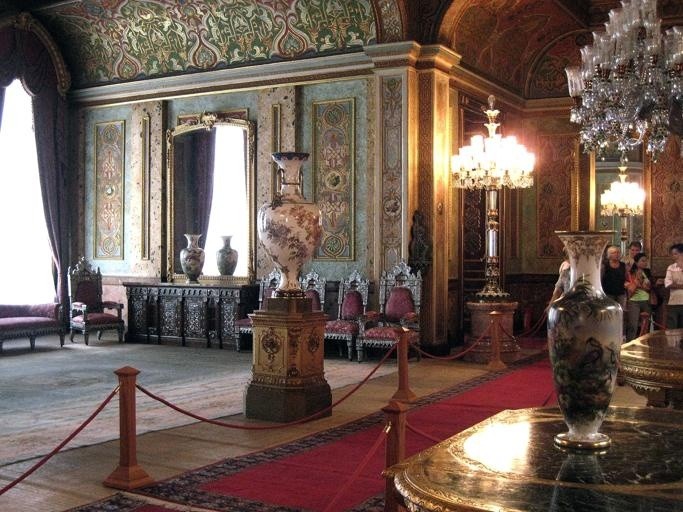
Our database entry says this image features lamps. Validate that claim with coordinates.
[598,166,648,257]
[447,93,538,303]
[562,0,682,167]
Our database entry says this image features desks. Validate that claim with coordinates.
[376,401,681,512]
[120,281,260,354]
[615,326,682,411]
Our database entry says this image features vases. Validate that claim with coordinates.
[180,232,206,285]
[255,151,324,298]
[545,229,626,457]
[216,235,238,275]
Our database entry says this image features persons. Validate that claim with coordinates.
[542,246,571,310]
[599,240,682,342]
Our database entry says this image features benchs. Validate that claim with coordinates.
[0,301,70,357]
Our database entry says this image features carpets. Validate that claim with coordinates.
[0,328,424,467]
[57,337,558,512]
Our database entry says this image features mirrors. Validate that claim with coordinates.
[586,127,653,270]
[163,106,258,285]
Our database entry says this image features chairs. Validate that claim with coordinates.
[65,254,125,346]
[231,256,424,365]
